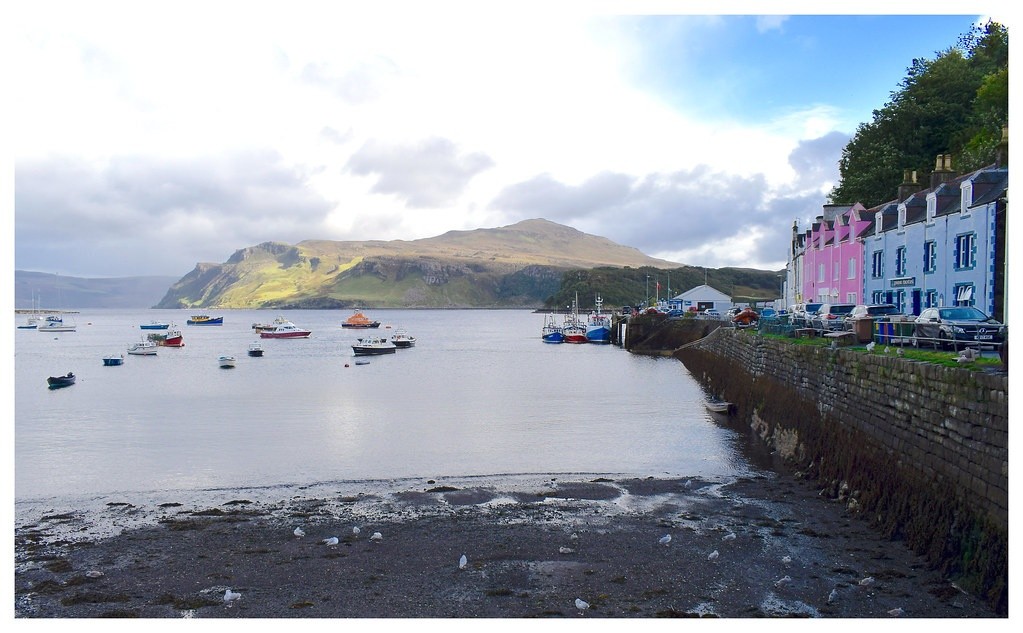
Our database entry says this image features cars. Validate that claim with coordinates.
[727,303,919,346]
[621,305,722,320]
[912,305,1005,351]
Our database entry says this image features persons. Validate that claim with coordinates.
[734,306,741,315]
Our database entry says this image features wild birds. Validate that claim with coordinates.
[86,570,101,577]
[353,526,360,538]
[370,532,383,542]
[828,589,839,602]
[775,555,792,587]
[459,555,467,569]
[224,590,241,608]
[323,537,338,549]
[722,533,737,541]
[888,608,904,616]
[659,534,671,546]
[560,547,571,553]
[575,599,589,615]
[685,480,692,489]
[707,550,718,560]
[858,577,875,586]
[865,341,904,358]
[294,527,305,539]
[571,533,579,544]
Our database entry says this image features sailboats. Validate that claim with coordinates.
[246,340,265,357]
[37,269,79,332]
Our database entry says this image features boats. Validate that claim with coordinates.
[187,314,224,326]
[218,356,236,368]
[350,335,397,355]
[17,286,65,329]
[126,333,158,356]
[340,299,381,329]
[139,320,170,329]
[391,320,417,348]
[102,353,126,365]
[147,320,186,347]
[251,312,312,339]
[540,288,612,345]
[46,371,77,388]
